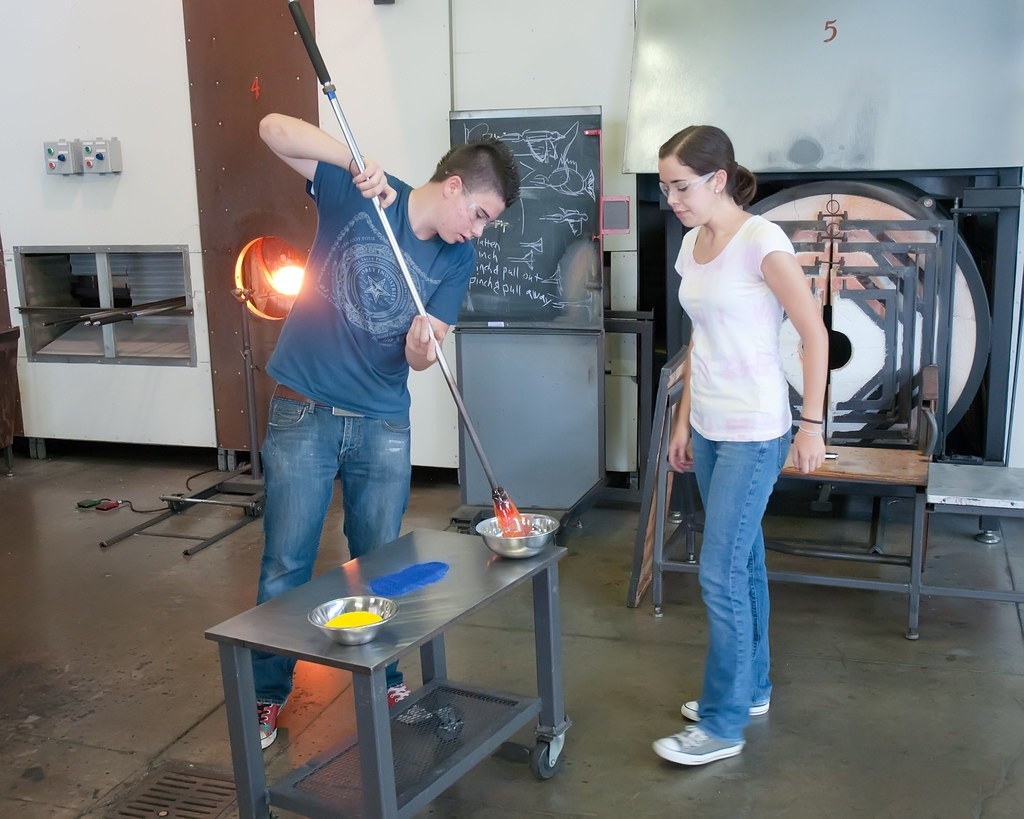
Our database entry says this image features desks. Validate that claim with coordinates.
[202,522,574,817]
[667,434,1024,651]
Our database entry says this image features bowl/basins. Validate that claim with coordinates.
[476,513,561,559]
[308,595,400,645]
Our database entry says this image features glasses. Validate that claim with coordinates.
[658,171,716,199]
[445,170,497,230]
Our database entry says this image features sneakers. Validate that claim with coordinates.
[387,683,432,724]
[653,725,746,766]
[256,702,284,750]
[680,700,770,723]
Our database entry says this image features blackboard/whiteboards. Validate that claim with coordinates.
[446,104,606,338]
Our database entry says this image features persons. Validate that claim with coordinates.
[651,123,831,767]
[251,110,522,751]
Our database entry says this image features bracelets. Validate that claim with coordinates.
[799,426,825,436]
[350,153,365,179]
[800,417,823,424]
